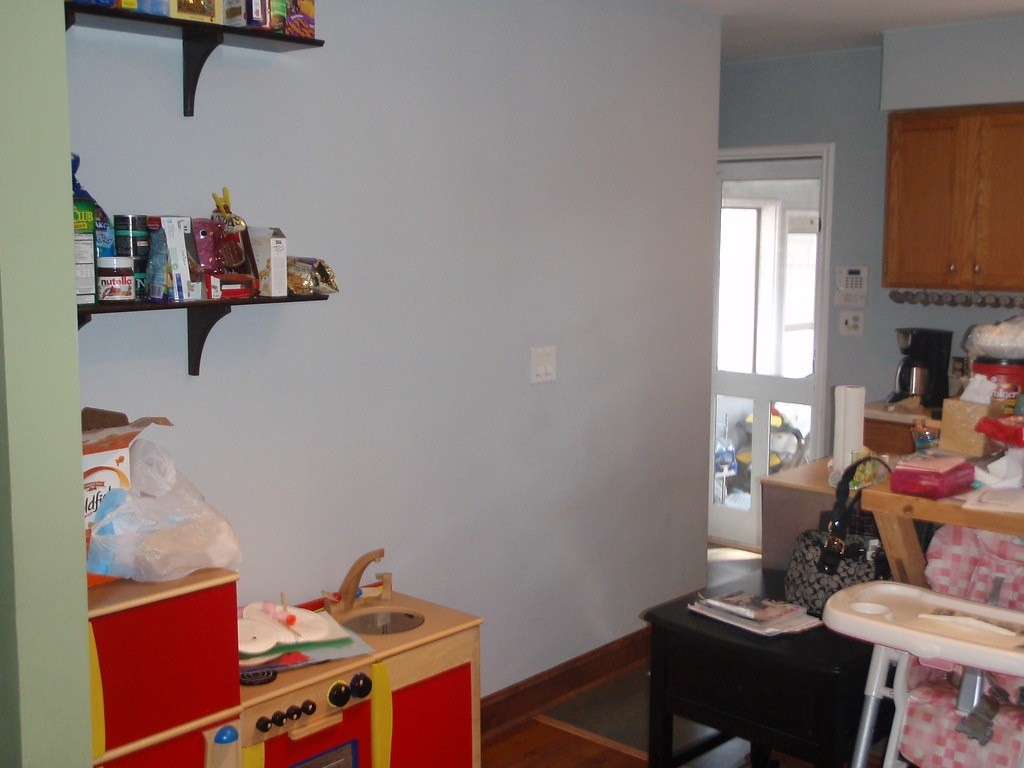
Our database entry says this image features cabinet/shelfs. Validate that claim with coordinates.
[880,102,1024,293]
[64,1,329,376]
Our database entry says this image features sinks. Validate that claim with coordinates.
[335,607,426,635]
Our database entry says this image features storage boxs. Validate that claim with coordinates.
[244,225,287,297]
[171,0,315,38]
[81,407,173,587]
[888,463,976,499]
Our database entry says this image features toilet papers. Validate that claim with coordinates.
[834,384,866,471]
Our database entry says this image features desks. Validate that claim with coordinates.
[639,563,896,768]
[759,457,838,577]
[864,400,938,456]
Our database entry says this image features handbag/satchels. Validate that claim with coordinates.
[784,454,895,617]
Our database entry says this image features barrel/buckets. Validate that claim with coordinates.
[972,356,1024,418]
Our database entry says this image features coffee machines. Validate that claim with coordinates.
[884,326,954,409]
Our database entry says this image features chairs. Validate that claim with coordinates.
[822,487,1023,768]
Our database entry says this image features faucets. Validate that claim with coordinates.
[321,548,386,615]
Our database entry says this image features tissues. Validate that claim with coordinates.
[938,373,1006,457]
